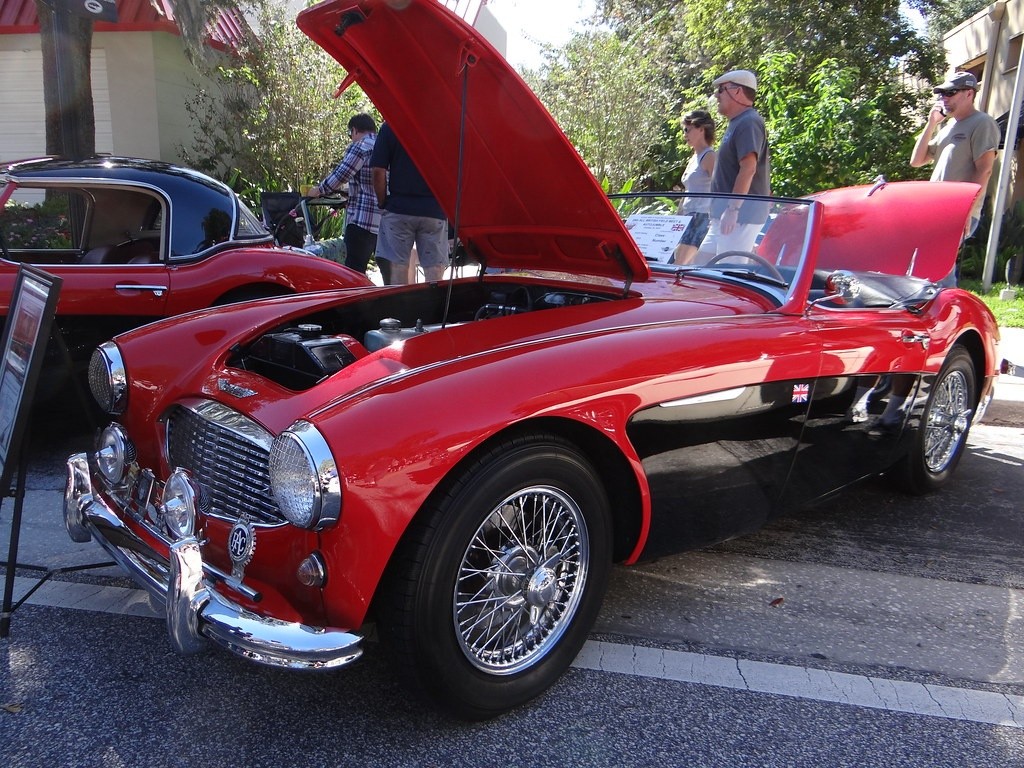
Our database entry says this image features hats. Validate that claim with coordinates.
[713,70,756,90]
[933,72,977,94]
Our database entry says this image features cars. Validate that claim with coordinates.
[63,0,999,720]
[0,153,379,354]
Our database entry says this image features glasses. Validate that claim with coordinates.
[715,86,738,94]
[348,127,355,138]
[683,126,695,134]
[941,89,958,97]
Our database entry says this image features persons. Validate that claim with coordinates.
[692,70,776,272]
[370,118,450,285]
[676,108,717,269]
[908,73,1003,289]
[307,115,392,285]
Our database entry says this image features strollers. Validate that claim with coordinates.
[260,191,348,257]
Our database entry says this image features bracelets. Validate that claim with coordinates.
[727,205,740,211]
[377,201,385,210]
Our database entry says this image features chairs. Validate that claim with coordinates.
[78,231,163,265]
